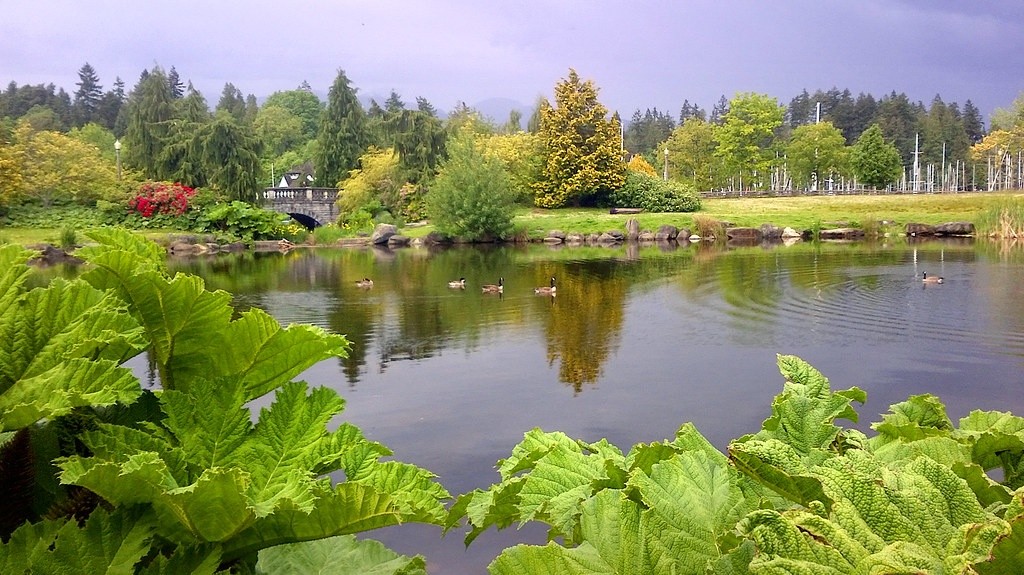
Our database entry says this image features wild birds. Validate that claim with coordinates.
[448,278,466,286]
[354,277,374,287]
[481,276,505,290]
[532,277,557,292]
[922,270,945,282]
